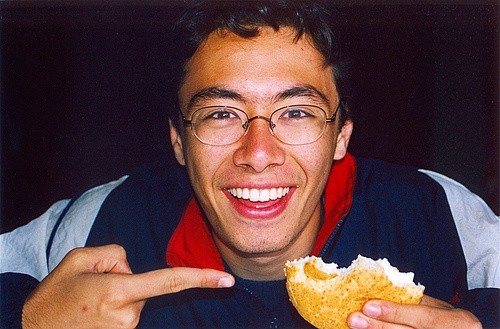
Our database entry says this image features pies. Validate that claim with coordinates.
[283,254,425,329]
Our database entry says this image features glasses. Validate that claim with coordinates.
[174,101,340,146]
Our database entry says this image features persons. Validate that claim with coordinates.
[0,1,500,329]
